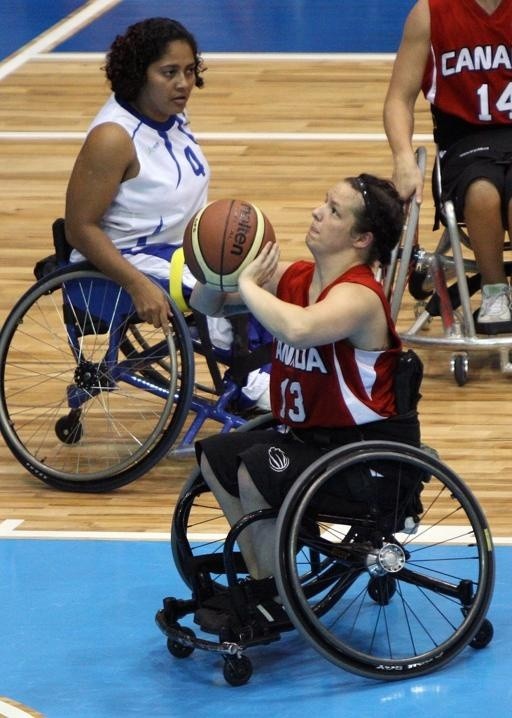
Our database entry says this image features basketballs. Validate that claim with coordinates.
[184,199,276,294]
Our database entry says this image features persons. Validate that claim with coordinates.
[64,17,272,414]
[383,0,512,335]
[189,175,402,634]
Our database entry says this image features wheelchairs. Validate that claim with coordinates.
[375,140,512,389]
[0,213,284,496]
[152,346,497,689]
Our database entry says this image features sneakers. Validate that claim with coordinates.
[476,283,512,335]
[194,574,284,633]
